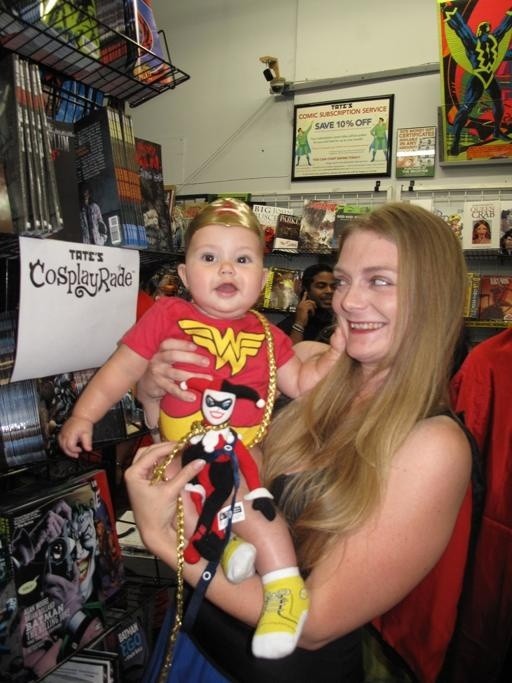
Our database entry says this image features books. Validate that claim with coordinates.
[1,0,176,257]
[419,198,511,323]
[0,295,151,682]
[176,193,380,314]
[142,255,194,304]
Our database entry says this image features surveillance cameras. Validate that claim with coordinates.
[263,68,277,82]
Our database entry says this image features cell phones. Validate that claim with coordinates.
[299,287,310,301]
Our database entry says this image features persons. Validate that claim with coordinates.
[55,197,345,663]
[275,263,337,344]
[120,200,478,681]
[448,321,512,683]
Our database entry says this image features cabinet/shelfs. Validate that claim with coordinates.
[171,185,512,361]
[0,0,193,683]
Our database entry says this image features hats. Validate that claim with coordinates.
[183,196,266,258]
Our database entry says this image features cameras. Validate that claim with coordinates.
[44,535,76,589]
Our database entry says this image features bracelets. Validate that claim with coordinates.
[140,408,160,437]
[290,321,304,336]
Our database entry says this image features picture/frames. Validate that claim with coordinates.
[291,93,395,181]
